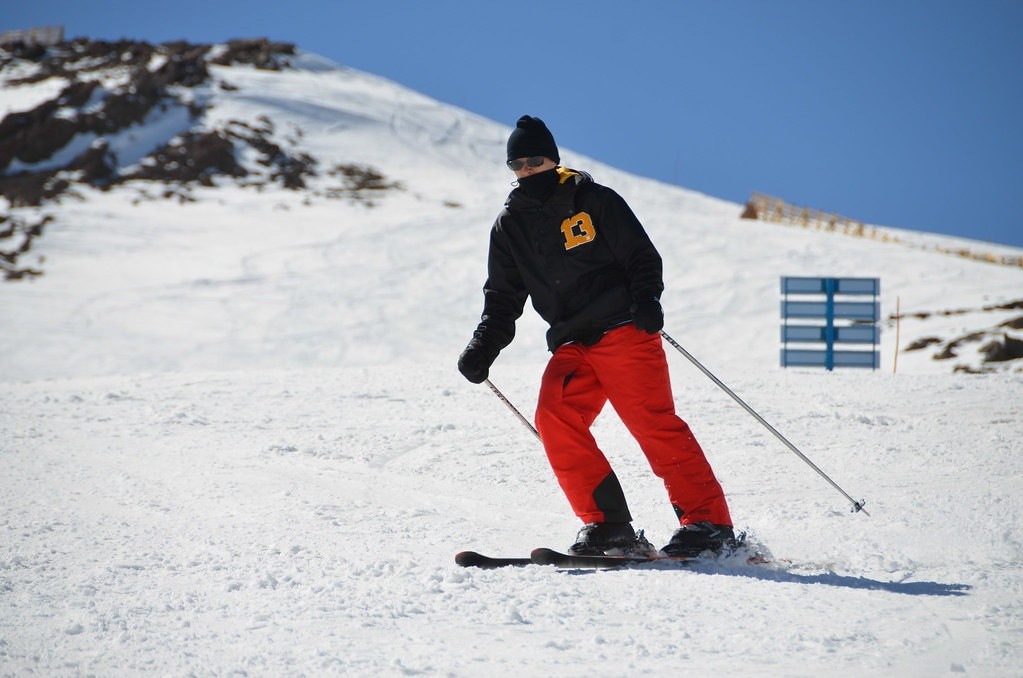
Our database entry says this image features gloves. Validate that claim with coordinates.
[458,349,488,383]
[629,298,663,333]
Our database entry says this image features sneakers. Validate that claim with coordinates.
[566,521,637,556]
[659,521,739,558]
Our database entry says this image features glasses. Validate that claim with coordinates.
[507,156,545,171]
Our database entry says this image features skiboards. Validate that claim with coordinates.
[454,547,792,570]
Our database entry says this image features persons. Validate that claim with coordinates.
[455,114,743,559]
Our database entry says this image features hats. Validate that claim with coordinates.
[507,114,560,164]
